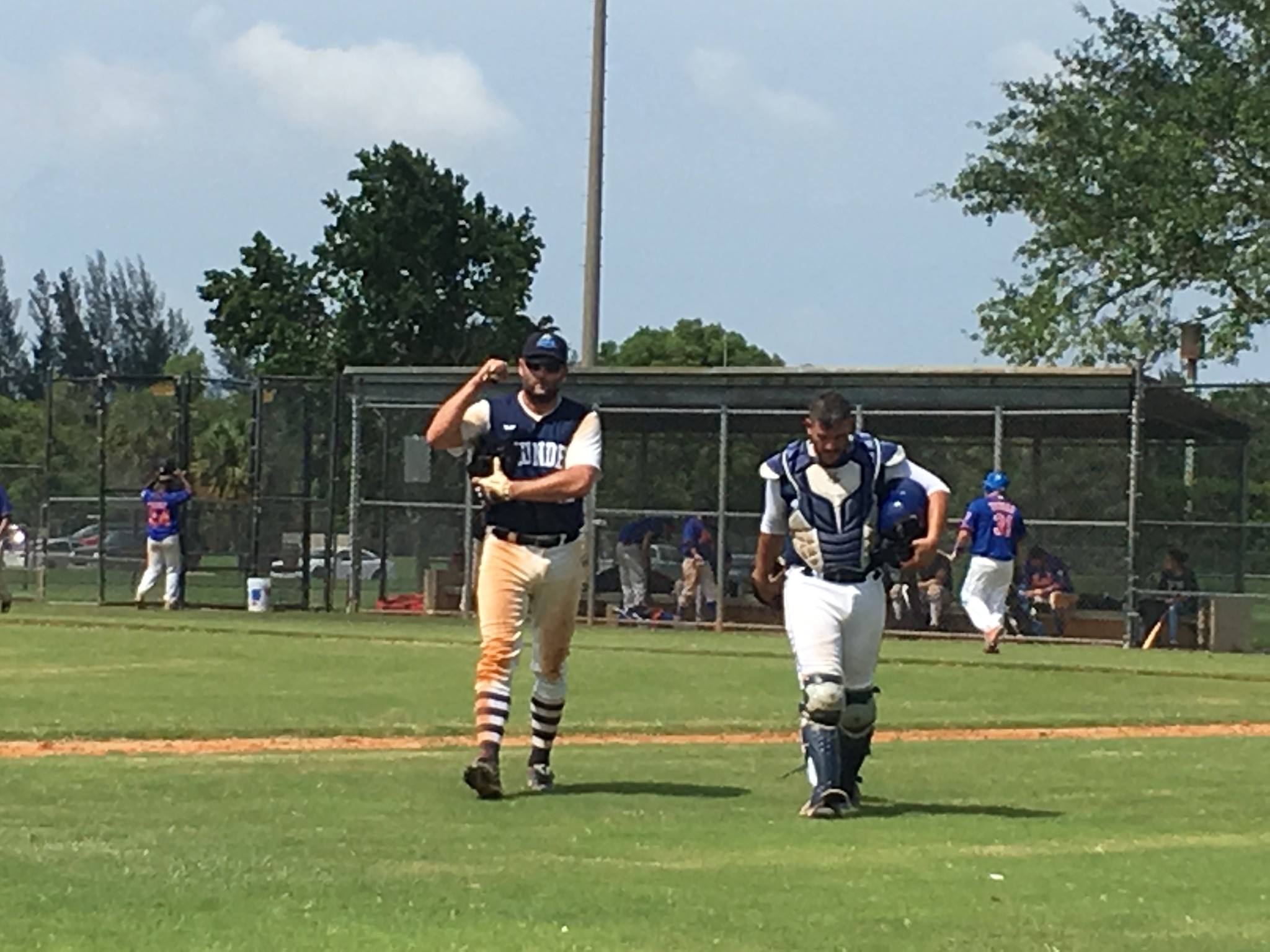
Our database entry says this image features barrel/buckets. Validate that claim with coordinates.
[247,578,271,613]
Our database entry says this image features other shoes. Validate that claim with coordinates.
[165,601,182,610]
[135,593,146,610]
[983,625,1005,654]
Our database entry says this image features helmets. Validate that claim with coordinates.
[983,471,1008,492]
[877,475,930,543]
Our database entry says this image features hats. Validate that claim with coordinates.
[518,332,569,364]
[157,467,173,482]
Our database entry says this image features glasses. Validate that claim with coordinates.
[523,356,564,374]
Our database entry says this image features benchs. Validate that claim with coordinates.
[441,579,1198,652]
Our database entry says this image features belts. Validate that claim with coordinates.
[491,526,580,548]
[802,565,881,584]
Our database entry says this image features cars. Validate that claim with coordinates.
[269,545,394,581]
[19,518,203,571]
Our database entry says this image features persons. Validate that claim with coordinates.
[1017,545,1074,637]
[891,552,953,632]
[426,333,600,799]
[754,391,951,815]
[1138,549,1201,646]
[133,463,193,609]
[674,501,717,622]
[615,516,674,618]
[948,472,1027,653]
[0,486,12,612]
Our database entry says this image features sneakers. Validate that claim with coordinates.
[811,791,845,819]
[464,757,503,800]
[525,762,556,793]
[842,780,860,815]
